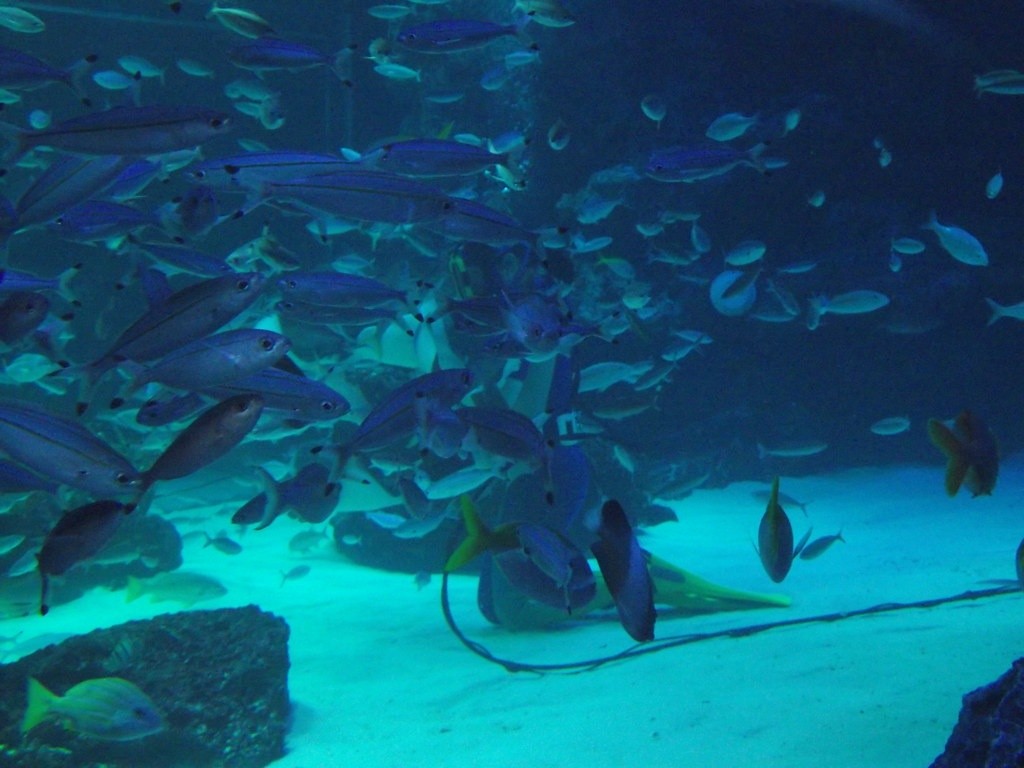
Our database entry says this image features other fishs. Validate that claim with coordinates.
[20,675,170,742]
[0,0,1024,644]
